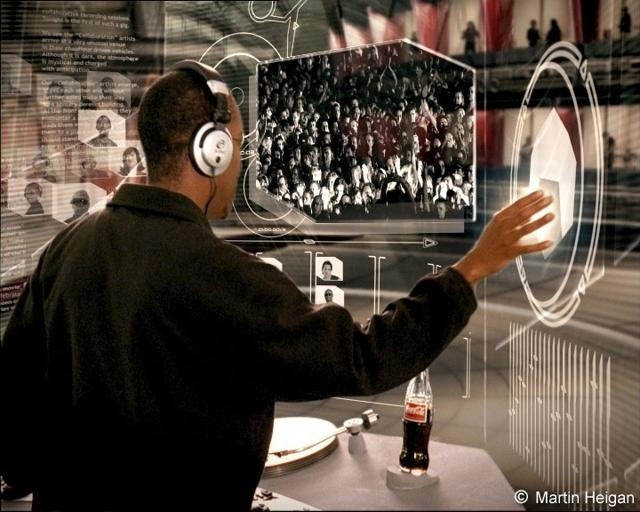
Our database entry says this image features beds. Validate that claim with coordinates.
[250,427,527,512]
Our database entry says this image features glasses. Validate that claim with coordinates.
[71,199,89,207]
[233,132,246,148]
[324,293,332,296]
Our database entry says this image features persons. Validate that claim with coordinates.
[40,77,75,111]
[546,17,564,47]
[118,149,144,175]
[87,115,119,149]
[320,261,339,281]
[0,58,555,511]
[463,22,482,55]
[96,76,129,116]
[324,289,334,302]
[253,43,476,221]
[66,190,90,224]
[602,130,616,168]
[26,153,58,185]
[526,17,541,46]
[24,183,44,215]
[81,157,118,190]
[520,134,534,163]
[617,5,633,38]
[0,59,25,97]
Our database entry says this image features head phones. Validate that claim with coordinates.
[166,60,234,177]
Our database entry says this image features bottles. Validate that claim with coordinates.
[399,368,432,475]
